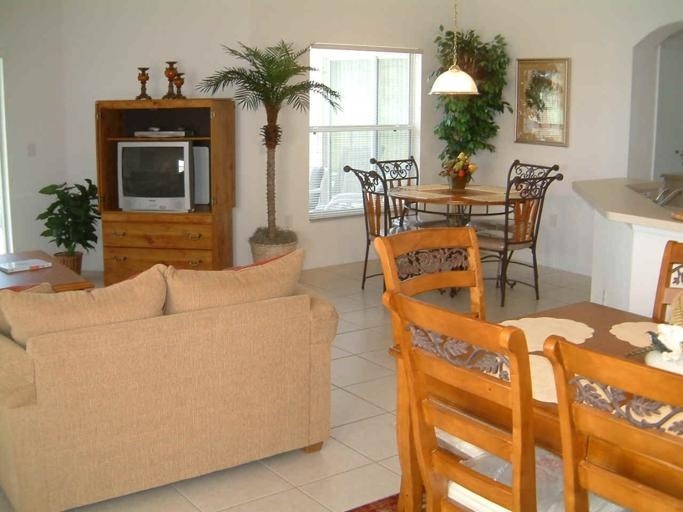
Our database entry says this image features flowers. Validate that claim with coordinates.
[438,152,477,184]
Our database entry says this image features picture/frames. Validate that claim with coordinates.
[515,57,571,148]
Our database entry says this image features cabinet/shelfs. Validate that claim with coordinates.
[96,97,237,288]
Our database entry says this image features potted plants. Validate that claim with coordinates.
[196,38,344,266]
[35,177,102,275]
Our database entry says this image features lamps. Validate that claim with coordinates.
[429,62,482,96]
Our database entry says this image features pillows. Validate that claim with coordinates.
[0,266,166,348]
[158,247,305,316]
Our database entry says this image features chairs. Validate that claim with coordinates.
[476,174,564,307]
[472,158,560,287]
[372,225,485,349]
[369,155,447,228]
[545,333,683,512]
[343,166,424,291]
[651,240,683,336]
[380,290,539,512]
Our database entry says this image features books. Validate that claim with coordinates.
[1,258,51,273]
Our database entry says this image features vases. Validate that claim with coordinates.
[450,176,468,194]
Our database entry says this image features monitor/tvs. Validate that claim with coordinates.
[118,141,195,214]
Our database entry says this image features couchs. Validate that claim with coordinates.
[0,285,338,512]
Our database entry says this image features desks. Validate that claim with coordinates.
[0,250,95,293]
[371,183,543,299]
